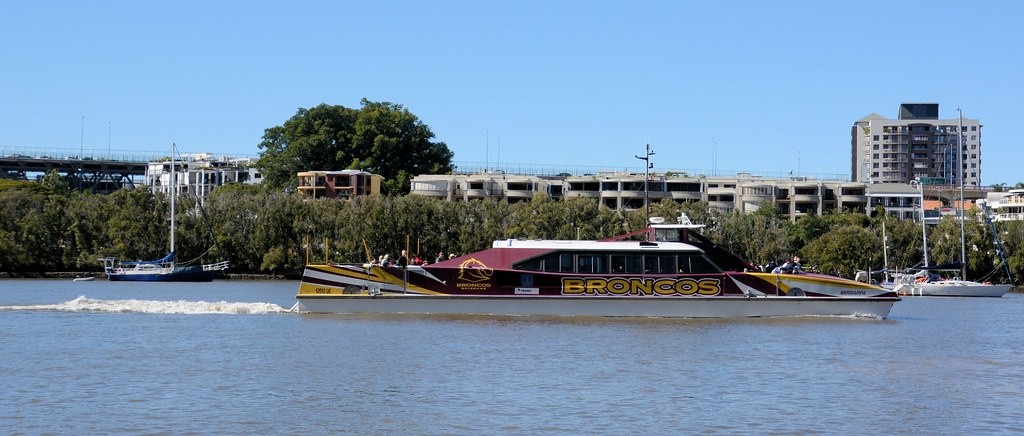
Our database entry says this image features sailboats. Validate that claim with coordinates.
[96,142,233,282]
[853,106,1017,297]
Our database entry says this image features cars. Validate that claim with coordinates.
[40,156,52,160]
[81,156,93,161]
[556,172,571,177]
[4,153,21,159]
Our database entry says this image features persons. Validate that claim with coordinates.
[378,249,454,267]
[750,256,807,274]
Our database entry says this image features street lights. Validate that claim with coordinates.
[106,122,112,193]
[80,115,85,194]
[497,135,500,170]
[486,129,489,172]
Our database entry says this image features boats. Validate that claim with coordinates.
[295,144,903,317]
[73,276,96,282]
